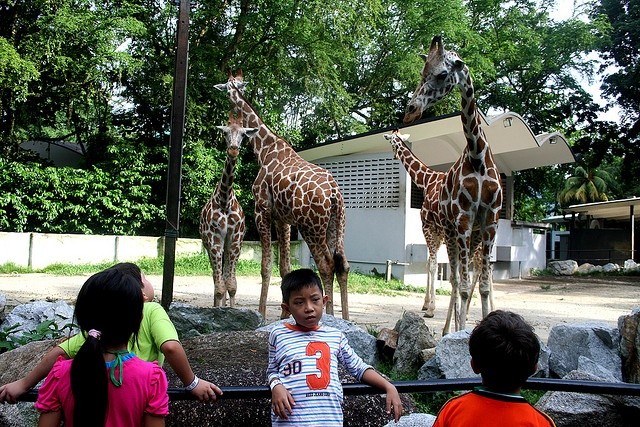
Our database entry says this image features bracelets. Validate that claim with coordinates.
[270,380,282,391]
[185,375,199,391]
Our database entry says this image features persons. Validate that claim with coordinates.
[33,270,170,426]
[265,269,403,427]
[432,310,558,426]
[0,262,224,405]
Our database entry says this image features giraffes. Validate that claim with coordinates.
[404,34,504,337]
[212,66,350,321]
[383,128,494,321]
[199,109,259,310]
